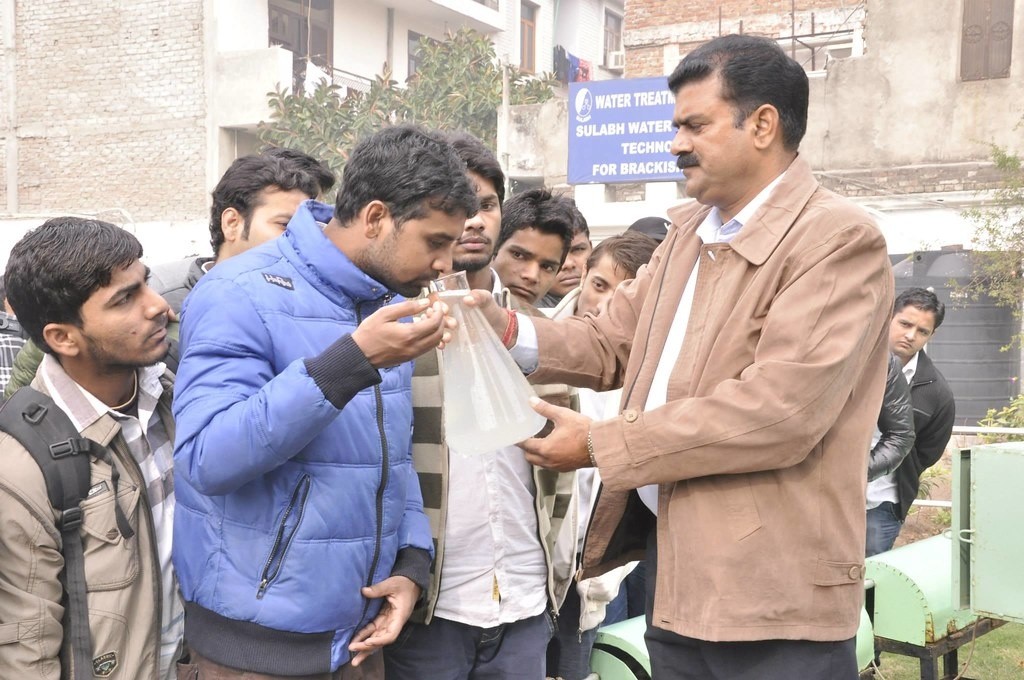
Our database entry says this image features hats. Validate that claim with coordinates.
[628,217,672,240]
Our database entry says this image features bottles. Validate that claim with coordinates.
[431,271,546,454]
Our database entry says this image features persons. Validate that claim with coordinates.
[0,123,672,680]
[420,34,895,680]
[865,287,956,667]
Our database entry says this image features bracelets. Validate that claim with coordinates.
[499,309,518,348]
[588,431,597,467]
